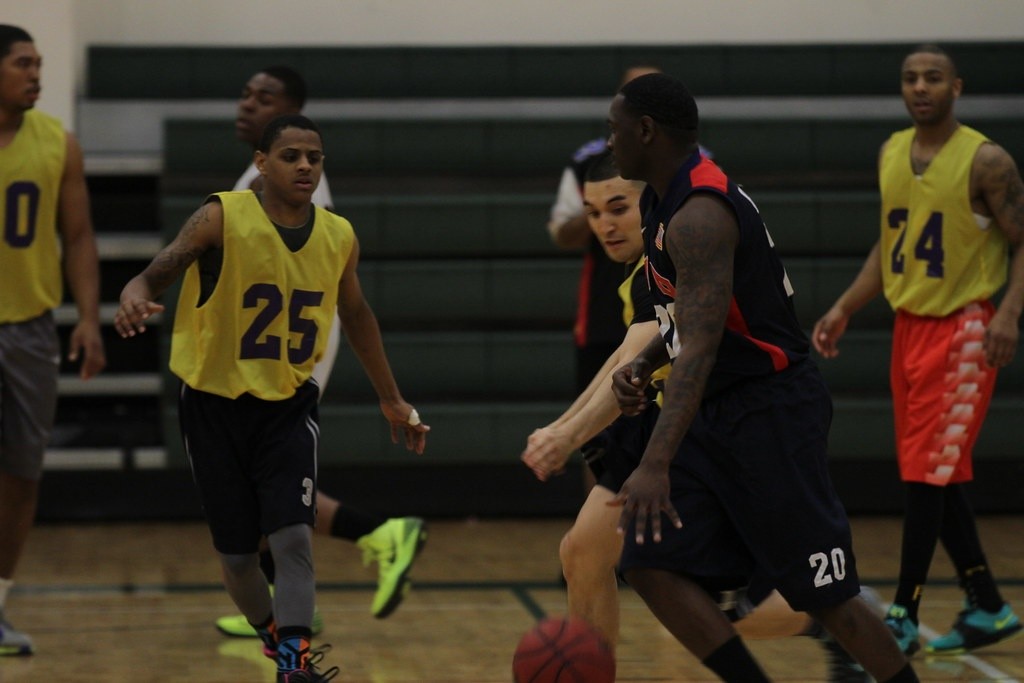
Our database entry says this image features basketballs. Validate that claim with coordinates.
[509,606,616,683]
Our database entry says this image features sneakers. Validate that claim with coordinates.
[276,666,339,683]
[214,585,323,637]
[355,517,426,617]
[885,606,921,655]
[924,600,1024,657]
[0,617,35,656]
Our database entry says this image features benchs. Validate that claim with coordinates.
[168,104,1022,476]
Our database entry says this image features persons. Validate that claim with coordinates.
[522,63,920,683]
[0,25,104,658]
[813,44,1023,655]
[110,59,431,682]
